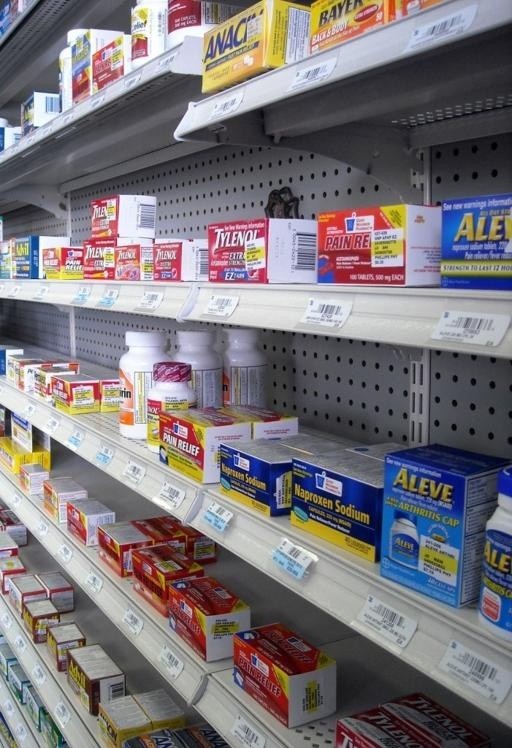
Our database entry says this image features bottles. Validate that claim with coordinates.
[58,27,89,114]
[129,0,168,70]
[475,462,512,633]
[170,328,225,413]
[219,327,271,409]
[117,330,172,442]
[144,360,199,457]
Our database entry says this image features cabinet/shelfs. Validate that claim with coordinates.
[0,2,511,747]
